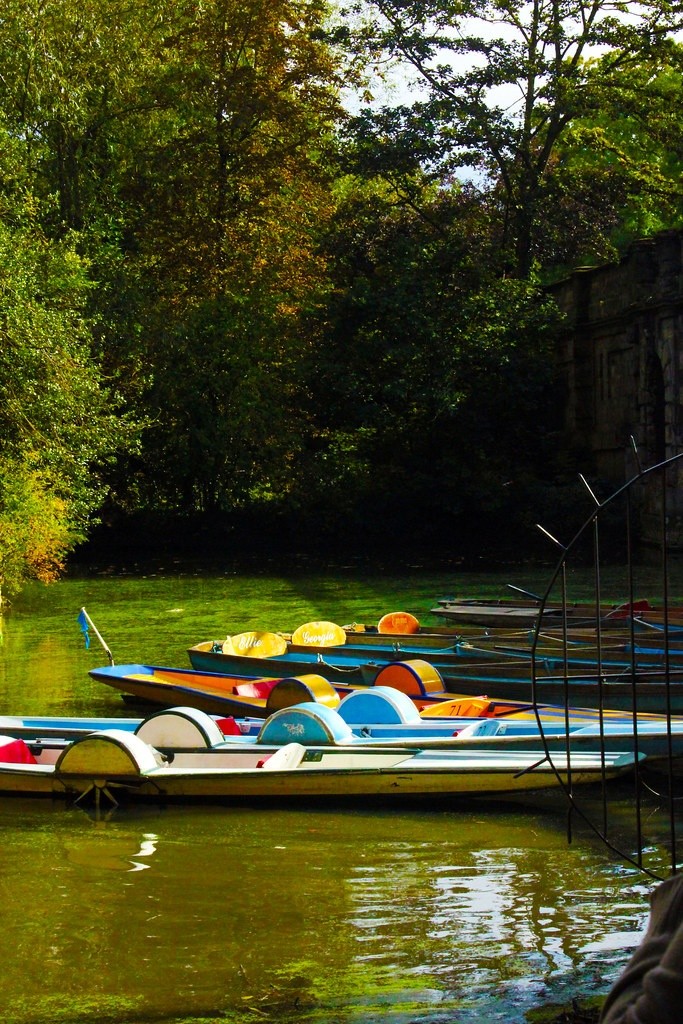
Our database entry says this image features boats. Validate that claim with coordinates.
[88,662,683,727]
[184,623,683,712]
[430,598,683,629]
[0,716,683,800]
